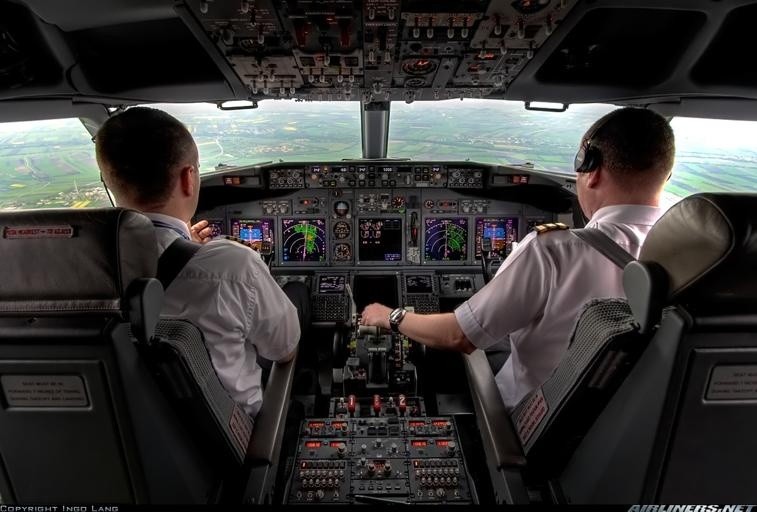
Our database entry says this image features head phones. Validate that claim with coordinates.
[574,110,618,175]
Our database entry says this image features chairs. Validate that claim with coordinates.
[456,186,757,511]
[0,206,294,512]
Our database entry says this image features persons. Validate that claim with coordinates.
[95,107,309,419]
[360,108,675,417]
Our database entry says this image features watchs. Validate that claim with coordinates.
[390,307,405,336]
